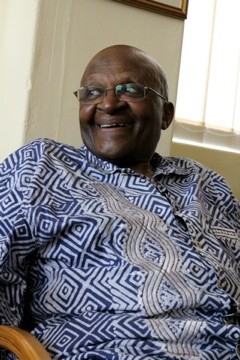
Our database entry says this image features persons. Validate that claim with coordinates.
[0,44,239,360]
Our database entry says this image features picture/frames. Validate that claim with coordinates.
[111,0,192,21]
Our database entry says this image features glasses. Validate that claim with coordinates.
[74,83,166,104]
